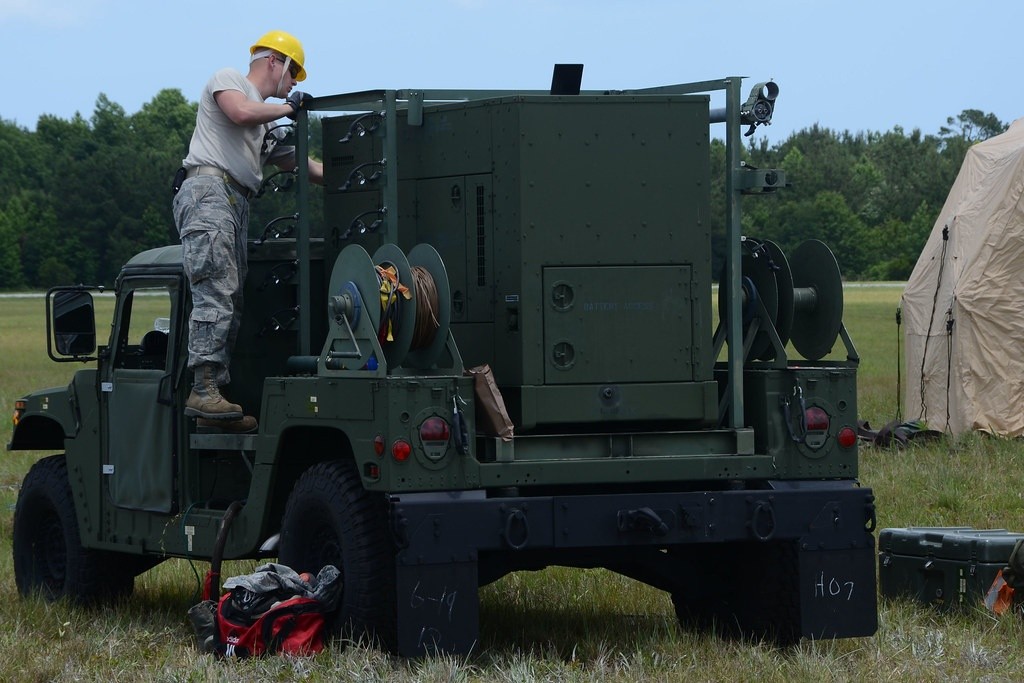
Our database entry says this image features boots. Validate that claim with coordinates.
[185,366,243,422]
[197,416,259,434]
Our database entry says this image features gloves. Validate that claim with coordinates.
[283,91,313,120]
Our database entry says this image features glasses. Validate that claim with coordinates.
[264,56,298,79]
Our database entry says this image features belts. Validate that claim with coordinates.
[186,166,253,202]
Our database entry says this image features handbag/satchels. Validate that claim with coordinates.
[463,363,514,442]
[214,590,331,665]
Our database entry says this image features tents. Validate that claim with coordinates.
[897,117,1024,439]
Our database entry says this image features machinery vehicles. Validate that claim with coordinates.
[7,62,882,664]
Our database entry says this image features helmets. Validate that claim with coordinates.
[249,30,307,82]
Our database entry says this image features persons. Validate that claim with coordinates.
[172,30,324,431]
